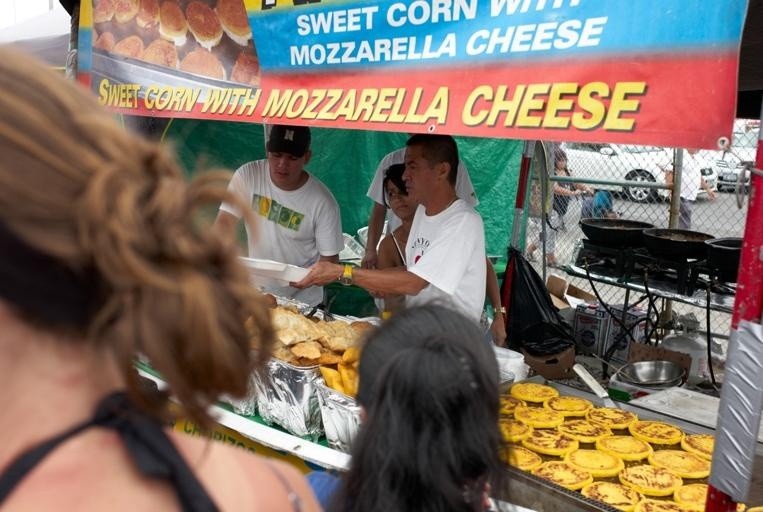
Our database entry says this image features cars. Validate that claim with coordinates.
[559,141,718,203]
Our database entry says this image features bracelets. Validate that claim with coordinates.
[492,306,508,320]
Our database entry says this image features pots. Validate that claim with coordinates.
[704,235,744,283]
[577,216,655,246]
[640,227,715,260]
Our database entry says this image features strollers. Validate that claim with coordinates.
[571,189,614,262]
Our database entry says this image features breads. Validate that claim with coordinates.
[320,346,360,397]
[245,294,376,368]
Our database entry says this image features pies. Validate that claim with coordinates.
[490,382,761,510]
[93,0,262,86]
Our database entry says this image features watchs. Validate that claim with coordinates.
[340,264,352,287]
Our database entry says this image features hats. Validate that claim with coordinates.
[266,125,310,158]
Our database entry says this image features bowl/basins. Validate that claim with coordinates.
[613,361,689,391]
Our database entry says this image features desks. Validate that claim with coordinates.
[558,259,737,381]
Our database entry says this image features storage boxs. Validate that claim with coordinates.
[545,272,654,364]
[523,344,578,382]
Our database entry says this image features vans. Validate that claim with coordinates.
[713,131,758,194]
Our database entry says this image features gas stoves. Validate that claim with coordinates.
[574,238,739,296]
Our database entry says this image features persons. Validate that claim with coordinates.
[524,150,591,270]
[302,305,516,511]
[603,209,617,221]
[366,162,509,347]
[361,132,481,269]
[662,147,717,231]
[0,46,327,511]
[201,123,348,314]
[286,130,488,329]
